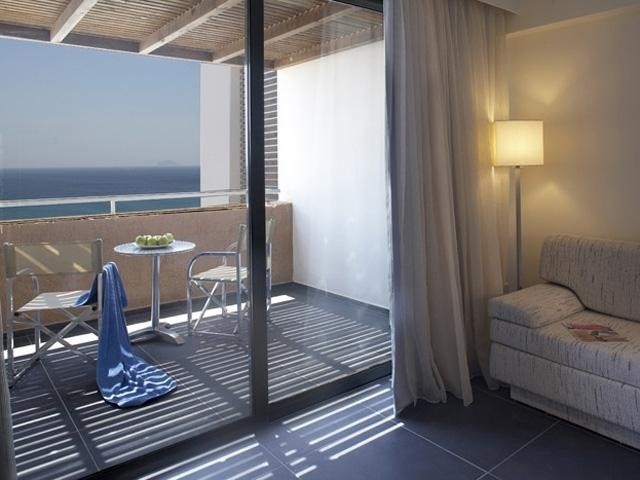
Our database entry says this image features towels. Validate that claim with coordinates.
[73,261,177,409]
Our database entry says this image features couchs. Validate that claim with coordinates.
[488,232,640,451]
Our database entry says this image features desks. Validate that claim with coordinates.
[112,241,194,345]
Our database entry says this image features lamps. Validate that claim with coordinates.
[489,121,544,290]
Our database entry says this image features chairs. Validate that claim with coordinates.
[3,239,104,388]
[186,218,271,338]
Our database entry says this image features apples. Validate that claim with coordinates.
[135,233,174,246]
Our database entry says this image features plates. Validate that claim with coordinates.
[132,240,175,249]
